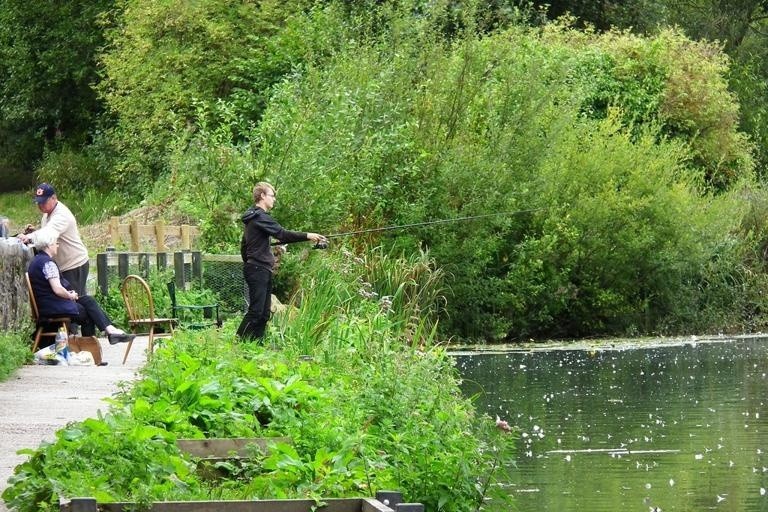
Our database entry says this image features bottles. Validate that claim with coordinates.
[55,327,68,361]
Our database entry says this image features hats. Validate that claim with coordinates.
[35,183,54,203]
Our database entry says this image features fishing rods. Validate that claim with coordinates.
[269,208,544,246]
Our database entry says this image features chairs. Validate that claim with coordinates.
[23,271,71,356]
[120,274,220,366]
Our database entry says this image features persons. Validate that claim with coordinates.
[237,181,328,347]
[17,184,90,335]
[28,238,137,366]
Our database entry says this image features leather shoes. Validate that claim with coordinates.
[108,332,136,345]
